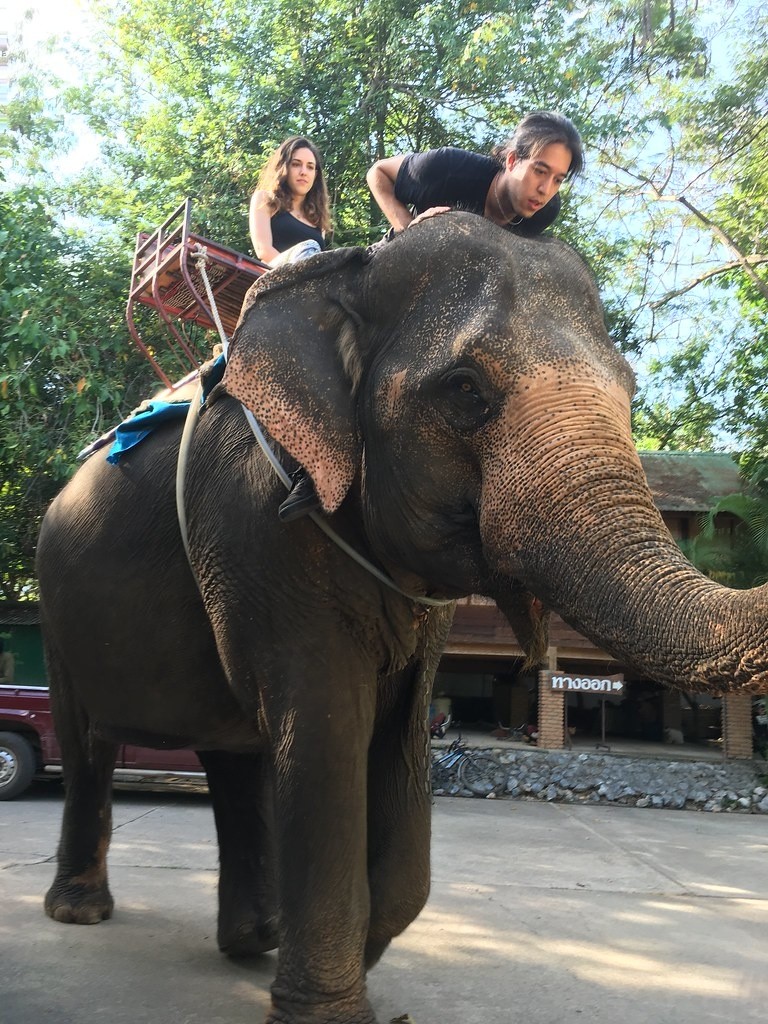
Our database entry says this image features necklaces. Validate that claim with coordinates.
[493,168,524,225]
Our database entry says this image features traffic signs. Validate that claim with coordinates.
[548,671,625,697]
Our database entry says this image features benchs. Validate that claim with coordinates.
[125,195,276,392]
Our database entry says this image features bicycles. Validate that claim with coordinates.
[424,725,508,795]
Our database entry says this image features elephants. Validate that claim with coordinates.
[34,210,768,1022]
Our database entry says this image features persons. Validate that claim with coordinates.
[365,111,585,240]
[250,136,334,267]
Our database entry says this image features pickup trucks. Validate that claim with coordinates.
[0,681,212,802]
